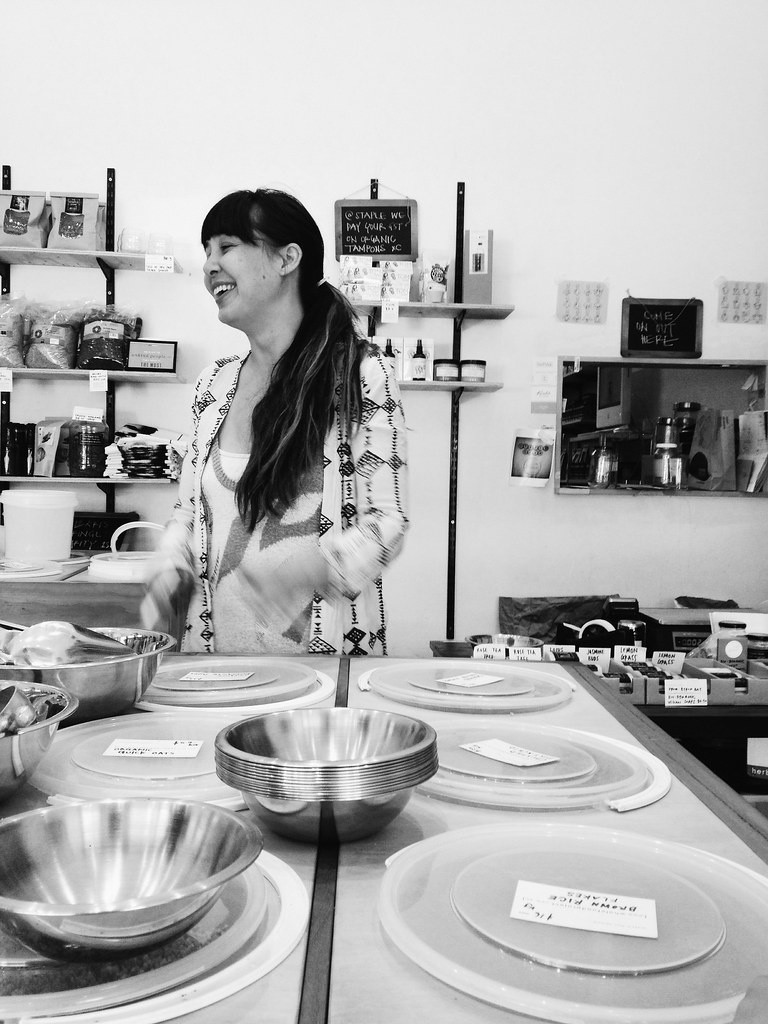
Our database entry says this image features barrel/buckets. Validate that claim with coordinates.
[0,490,78,559]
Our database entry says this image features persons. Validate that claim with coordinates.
[144,189,409,660]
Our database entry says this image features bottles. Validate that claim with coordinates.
[655,417,680,447]
[384,339,395,374]
[673,402,701,433]
[413,340,426,381]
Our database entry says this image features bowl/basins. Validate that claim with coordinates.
[0,798,263,961]
[465,635,544,655]
[0,680,79,802]
[216,706,439,842]
[0,628,176,721]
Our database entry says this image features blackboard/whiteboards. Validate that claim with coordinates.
[71,510,140,555]
[619,298,704,358]
[335,198,420,263]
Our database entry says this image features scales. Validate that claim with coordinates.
[633,608,756,659]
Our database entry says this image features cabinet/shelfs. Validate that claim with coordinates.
[330,179,516,639]
[0,162,188,630]
